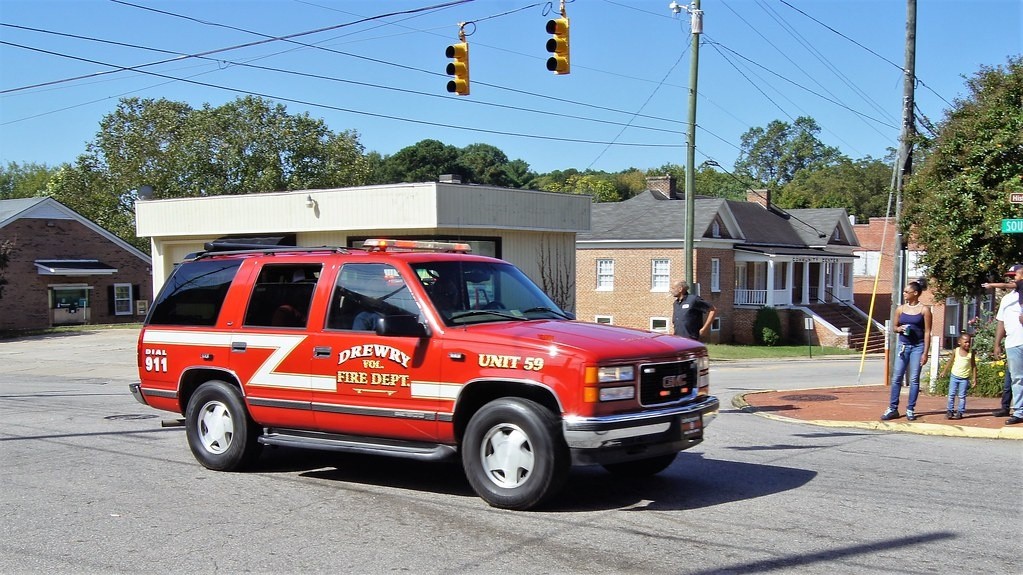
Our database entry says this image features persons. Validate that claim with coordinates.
[939,333,977,419]
[981,264,1023,425]
[669,279,716,342]
[881,279,932,420]
[352,276,386,330]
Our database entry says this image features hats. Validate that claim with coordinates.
[1004,266,1023,277]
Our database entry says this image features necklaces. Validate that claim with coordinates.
[908,301,920,307]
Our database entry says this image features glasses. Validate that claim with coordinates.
[903,291,914,294]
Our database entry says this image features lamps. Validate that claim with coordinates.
[306,194,315,208]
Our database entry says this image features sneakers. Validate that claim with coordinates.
[906,409,914,421]
[880,407,900,420]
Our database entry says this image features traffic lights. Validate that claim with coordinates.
[545,18,570,75]
[445,42,470,96]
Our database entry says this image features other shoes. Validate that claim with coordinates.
[1005,416,1023,424]
[945,410,954,418]
[993,409,1010,417]
[955,411,963,419]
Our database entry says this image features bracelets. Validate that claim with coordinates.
[923,353,927,355]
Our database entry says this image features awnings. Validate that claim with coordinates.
[213,236,296,246]
[35,260,118,276]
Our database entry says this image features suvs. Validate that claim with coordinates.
[131,238,719,509]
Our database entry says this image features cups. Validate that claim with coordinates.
[903,324,911,336]
